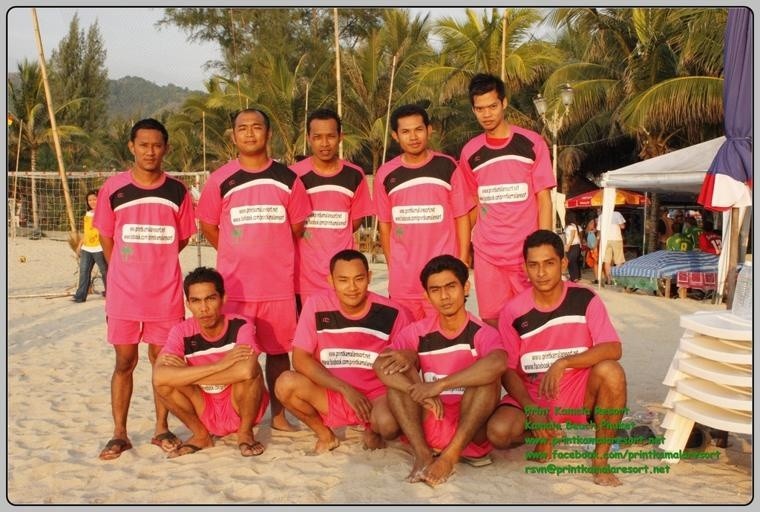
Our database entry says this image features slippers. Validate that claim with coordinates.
[151,432,183,451]
[167,441,199,458]
[434,448,492,467]
[239,442,265,457]
[100,439,132,460]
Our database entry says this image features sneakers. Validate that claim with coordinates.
[592,278,613,287]
[630,425,656,446]
[613,428,632,449]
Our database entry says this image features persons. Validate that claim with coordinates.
[92,118,199,462]
[371,102,477,322]
[560,196,724,300]
[66,188,109,304]
[197,106,315,435]
[274,249,420,457]
[484,226,630,491]
[286,109,373,322]
[153,265,271,457]
[373,253,510,488]
[459,70,560,329]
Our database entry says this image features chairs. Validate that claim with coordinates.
[655,253,753,464]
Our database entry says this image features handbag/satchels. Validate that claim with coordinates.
[581,239,587,256]
[587,230,598,249]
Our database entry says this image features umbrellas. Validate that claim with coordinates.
[698,6,755,315]
[564,179,653,212]
